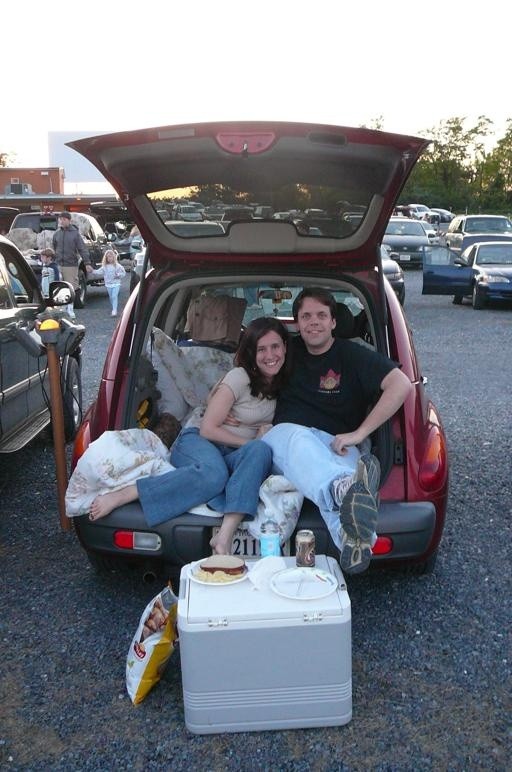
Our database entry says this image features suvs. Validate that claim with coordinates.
[0,238,86,460]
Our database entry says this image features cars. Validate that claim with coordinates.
[65,124,452,584]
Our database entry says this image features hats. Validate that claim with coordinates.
[58,213,71,220]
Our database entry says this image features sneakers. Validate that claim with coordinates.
[333,455,381,576]
[67,308,76,318]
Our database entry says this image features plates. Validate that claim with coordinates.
[266,566,339,602]
[188,557,251,587]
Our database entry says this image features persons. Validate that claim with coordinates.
[88,250,126,316]
[52,212,93,318]
[89,317,294,555]
[41,249,61,297]
[206,288,412,574]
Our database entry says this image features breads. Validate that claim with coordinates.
[200,554,246,575]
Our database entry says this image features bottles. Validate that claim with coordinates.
[259,507,281,557]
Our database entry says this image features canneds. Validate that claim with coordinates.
[294,529,316,568]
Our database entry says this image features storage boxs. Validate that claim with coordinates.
[175,554,354,736]
